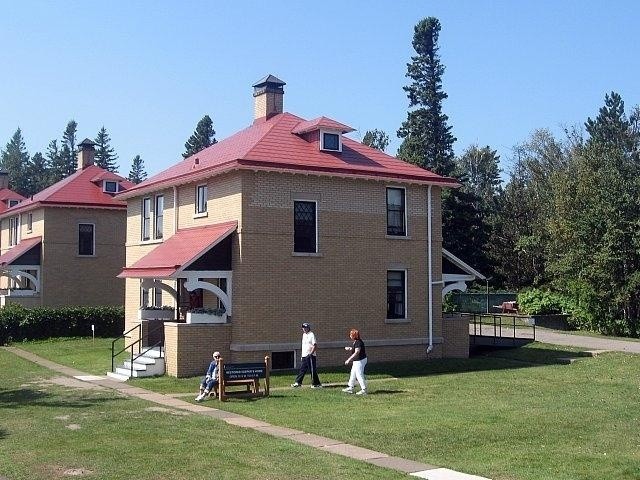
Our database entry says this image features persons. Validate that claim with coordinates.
[291,323,322,389]
[187,292,199,309]
[195,351,221,402]
[342,328,368,394]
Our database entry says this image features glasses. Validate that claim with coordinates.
[214,356,220,358]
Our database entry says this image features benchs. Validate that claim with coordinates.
[214,356,271,400]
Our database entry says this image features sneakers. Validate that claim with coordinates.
[356,389,367,395]
[342,388,353,393]
[195,395,204,402]
[310,385,319,388]
[290,383,300,387]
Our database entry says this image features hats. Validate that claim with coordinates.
[301,323,310,328]
